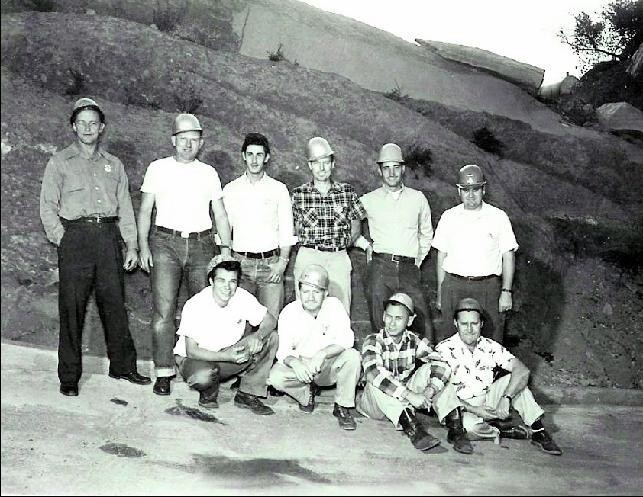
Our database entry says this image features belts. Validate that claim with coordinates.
[301,242,348,253]
[444,271,499,282]
[231,248,281,259]
[372,251,415,265]
[63,215,120,226]
[153,224,212,240]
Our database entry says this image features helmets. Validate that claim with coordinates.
[455,296,484,314]
[455,164,487,190]
[69,97,105,132]
[205,252,239,277]
[382,292,416,318]
[374,142,409,165]
[298,263,330,291]
[171,114,203,136]
[306,136,335,162]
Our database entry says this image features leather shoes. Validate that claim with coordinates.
[152,375,171,396]
[107,367,151,386]
[197,386,219,410]
[59,380,79,397]
[233,391,275,416]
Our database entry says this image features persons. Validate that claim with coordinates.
[216,132,294,318]
[430,164,519,345]
[172,253,279,415]
[268,265,362,431]
[139,113,233,395]
[360,292,474,454]
[434,298,563,456]
[289,137,367,314]
[38,98,151,396]
[351,142,434,345]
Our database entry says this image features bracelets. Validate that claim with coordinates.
[255,334,263,341]
[220,246,232,250]
[501,395,512,403]
[278,257,290,263]
[425,386,436,394]
[502,288,512,292]
[404,390,412,400]
[126,248,137,251]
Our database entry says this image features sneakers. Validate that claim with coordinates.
[298,385,316,414]
[529,428,562,455]
[489,420,529,440]
[332,402,358,430]
[446,427,474,454]
[410,429,440,451]
